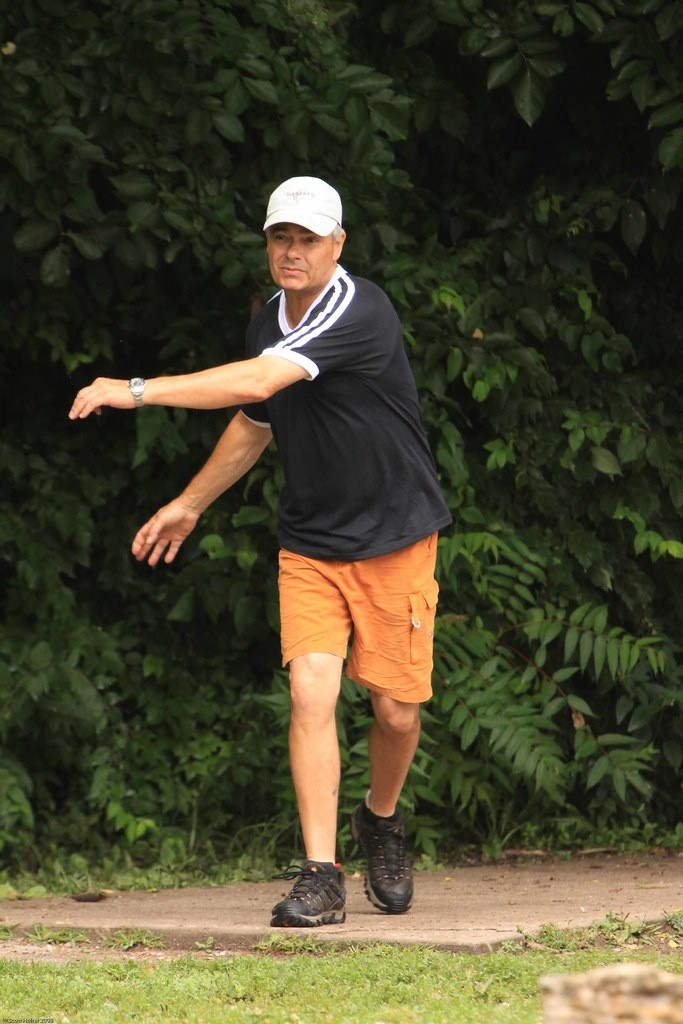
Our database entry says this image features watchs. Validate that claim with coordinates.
[127,377,146,408]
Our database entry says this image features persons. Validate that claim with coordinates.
[67,175,454,927]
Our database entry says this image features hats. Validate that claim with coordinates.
[263,178,342,236]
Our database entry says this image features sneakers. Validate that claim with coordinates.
[349,790,413,913]
[270,860,346,925]
